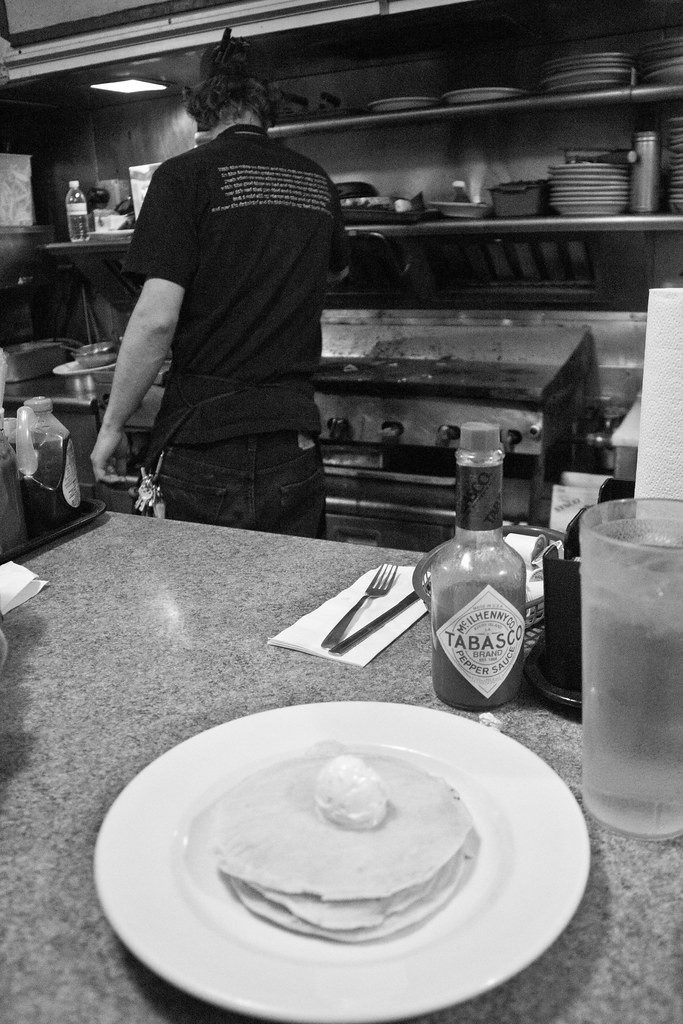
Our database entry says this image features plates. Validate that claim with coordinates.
[539,35,683,92]
[365,96,443,111]
[51,361,117,374]
[94,701,590,1022]
[427,201,491,218]
[443,87,530,104]
[546,163,630,217]
[85,230,135,243]
[666,117,683,215]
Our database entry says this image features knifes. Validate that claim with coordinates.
[328,582,431,656]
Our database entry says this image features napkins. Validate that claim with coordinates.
[0,559,50,616]
[267,564,432,668]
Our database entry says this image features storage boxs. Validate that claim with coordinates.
[2,339,66,383]
[487,179,545,219]
[128,161,167,223]
[0,153,36,228]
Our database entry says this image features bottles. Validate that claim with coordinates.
[65,180,89,243]
[0,395,80,555]
[429,423,526,712]
[629,119,661,215]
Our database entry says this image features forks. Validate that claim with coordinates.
[321,564,399,650]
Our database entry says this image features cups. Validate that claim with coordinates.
[580,498,683,841]
[93,208,119,232]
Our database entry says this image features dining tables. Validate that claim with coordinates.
[0,511,683,1024]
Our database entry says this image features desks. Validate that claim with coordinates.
[610,389,641,480]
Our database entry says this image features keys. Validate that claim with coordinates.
[134,466,163,511]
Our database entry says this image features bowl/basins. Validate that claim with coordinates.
[488,183,546,218]
[71,342,118,367]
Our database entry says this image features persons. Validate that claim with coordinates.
[87,68,353,538]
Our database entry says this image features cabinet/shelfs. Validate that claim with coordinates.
[193,80,683,239]
